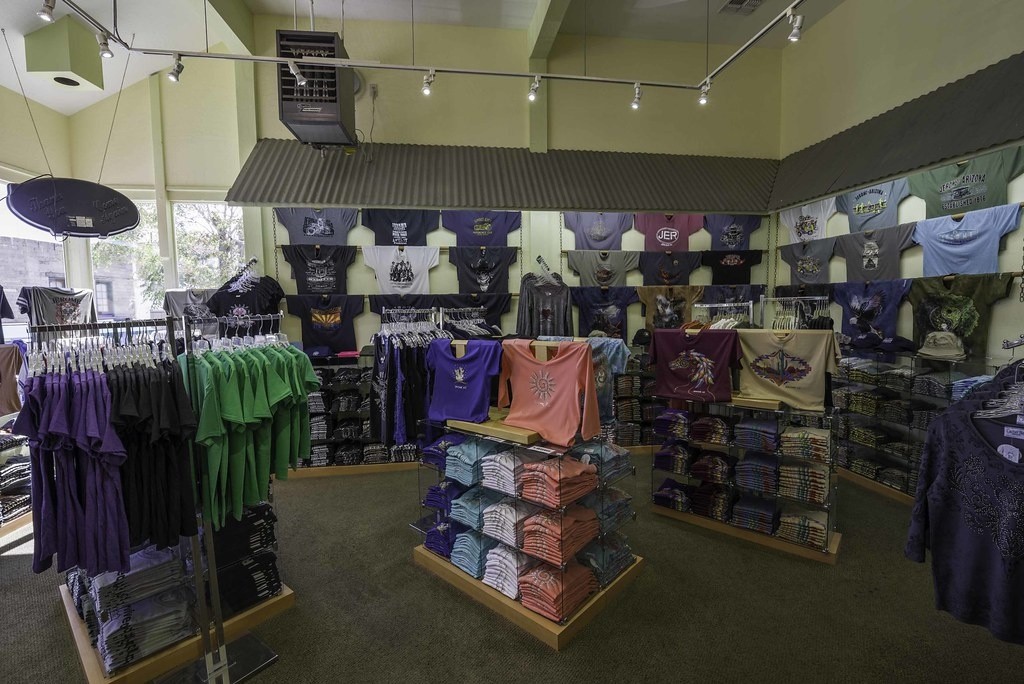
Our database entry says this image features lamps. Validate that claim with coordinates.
[526,76,542,101]
[36,0,57,22]
[786,9,805,42]
[421,70,435,96]
[96,30,115,58]
[286,61,307,86]
[630,83,643,109]
[167,54,185,82]
[698,76,713,105]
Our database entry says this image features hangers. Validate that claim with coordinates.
[191,313,290,359]
[971,348,1024,419]
[227,259,260,293]
[23,319,175,378]
[369,306,504,350]
[532,258,562,288]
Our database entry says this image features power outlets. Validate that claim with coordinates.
[370,84,378,96]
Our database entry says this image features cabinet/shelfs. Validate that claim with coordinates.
[0,447,33,538]
[831,348,1019,510]
[649,395,844,565]
[407,417,646,653]
[286,352,419,479]
[609,370,669,456]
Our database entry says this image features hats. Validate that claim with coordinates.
[875,335,918,352]
[848,333,881,348]
[918,331,964,355]
